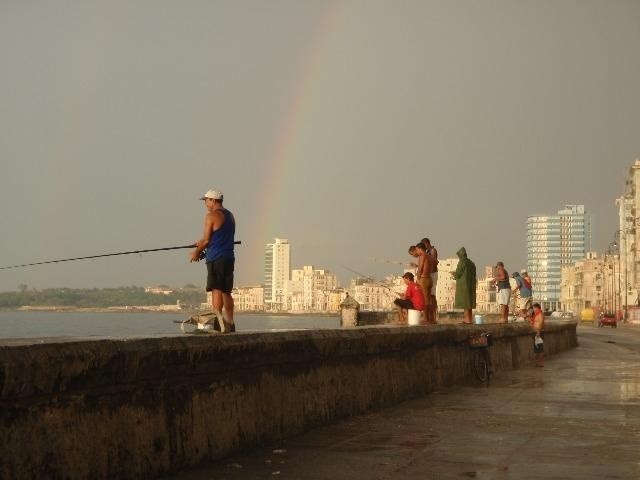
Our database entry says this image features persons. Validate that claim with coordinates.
[393,272,424,322]
[447,246,476,325]
[188,188,238,332]
[407,236,438,323]
[510,272,532,324]
[529,303,544,367]
[490,262,511,323]
[520,269,532,285]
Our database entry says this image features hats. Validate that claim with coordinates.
[199,188,223,204]
[520,269,527,274]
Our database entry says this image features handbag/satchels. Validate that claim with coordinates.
[523,279,532,289]
[534,335,544,346]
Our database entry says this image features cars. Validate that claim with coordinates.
[560,308,618,328]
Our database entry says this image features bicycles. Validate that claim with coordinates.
[469,331,494,383]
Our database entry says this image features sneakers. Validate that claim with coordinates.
[212,316,235,332]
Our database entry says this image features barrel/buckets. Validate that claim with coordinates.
[475,314,483,325]
[408,308,421,326]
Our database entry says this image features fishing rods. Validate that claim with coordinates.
[1,241,240,269]
[390,262,453,273]
[340,266,402,296]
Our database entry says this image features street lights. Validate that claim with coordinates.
[609,241,616,255]
